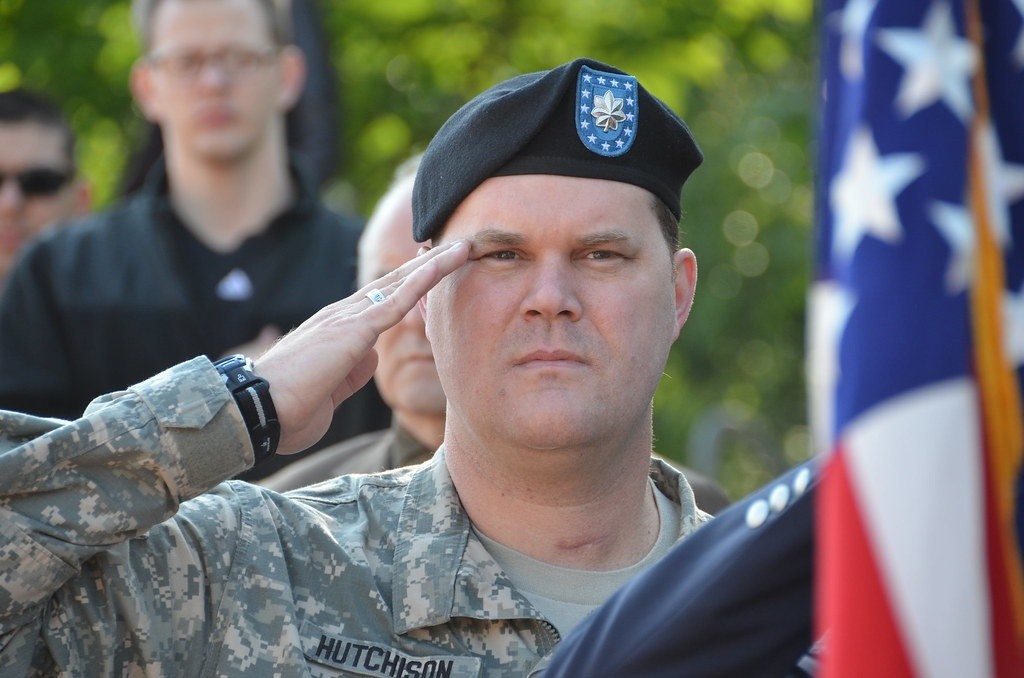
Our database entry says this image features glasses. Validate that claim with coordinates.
[0,166,76,198]
[146,43,279,72]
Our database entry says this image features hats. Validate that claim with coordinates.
[412,57,703,243]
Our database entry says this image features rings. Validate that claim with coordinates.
[364,288,386,306]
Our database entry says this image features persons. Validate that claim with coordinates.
[240,146,739,523]
[533,439,841,678]
[0,0,396,490]
[0,56,733,678]
[0,89,95,278]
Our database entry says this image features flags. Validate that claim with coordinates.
[808,0,1024,678]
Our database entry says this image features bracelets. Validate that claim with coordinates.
[214,354,280,465]
